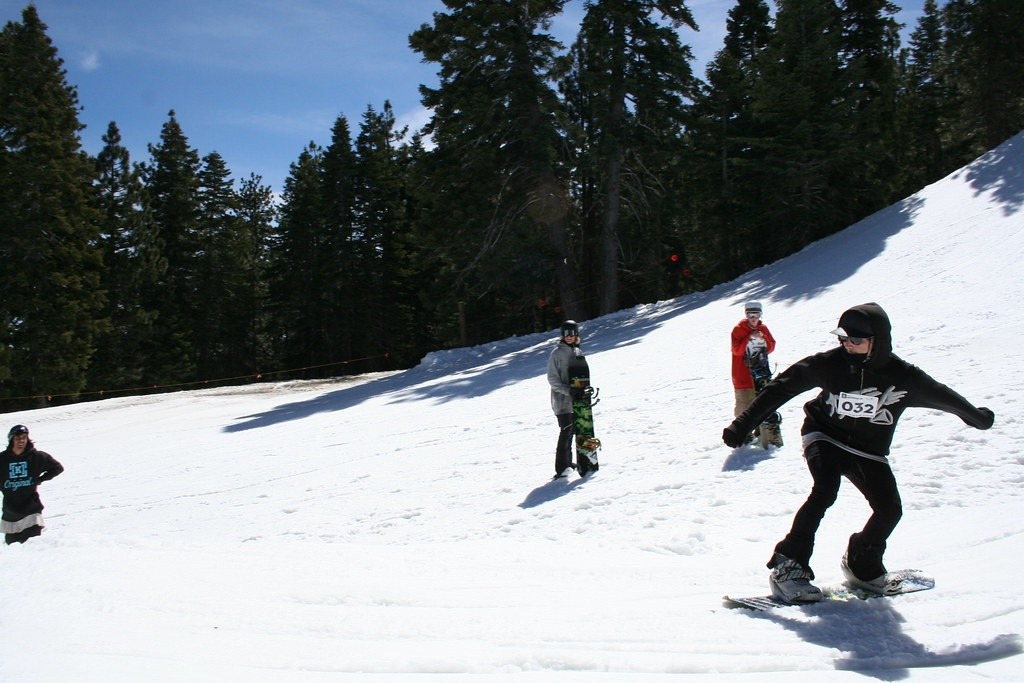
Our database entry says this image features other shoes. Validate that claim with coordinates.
[560,466,574,476]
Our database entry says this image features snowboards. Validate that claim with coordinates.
[721,566,937,614]
[566,349,600,480]
[743,333,786,451]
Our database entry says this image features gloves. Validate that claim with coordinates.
[570,388,583,401]
[722,424,747,449]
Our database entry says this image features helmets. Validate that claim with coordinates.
[744,300,762,313]
[561,320,579,336]
[7,425,29,441]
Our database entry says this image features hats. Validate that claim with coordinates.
[829,310,874,338]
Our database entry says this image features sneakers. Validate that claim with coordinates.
[840,547,904,595]
[765,551,823,605]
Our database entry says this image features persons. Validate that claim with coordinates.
[0,425,64,545]
[547,320,583,472]
[731,300,776,437]
[723,303,995,599]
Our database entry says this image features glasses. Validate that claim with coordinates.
[563,329,576,338]
[747,313,761,319]
[839,336,869,345]
